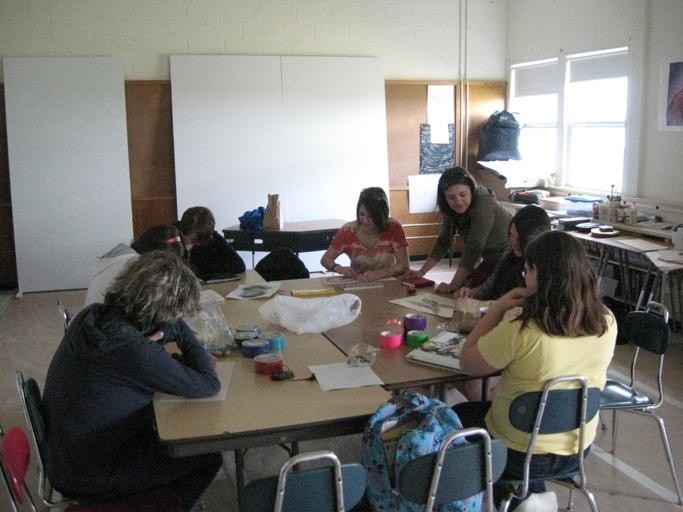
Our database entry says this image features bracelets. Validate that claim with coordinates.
[330,263,340,273]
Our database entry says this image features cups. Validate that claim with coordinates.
[592,197,638,224]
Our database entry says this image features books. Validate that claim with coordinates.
[405,328,473,377]
[579,238,682,323]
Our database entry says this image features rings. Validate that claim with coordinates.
[419,268,425,276]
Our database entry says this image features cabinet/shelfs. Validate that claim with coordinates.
[495,194,682,335]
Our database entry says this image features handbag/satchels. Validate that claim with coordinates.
[262,194,281,231]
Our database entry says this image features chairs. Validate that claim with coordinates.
[13,369,83,512]
[496,373,603,512]
[56,296,73,332]
[237,448,369,512]
[0,424,40,512]
[398,426,508,512]
[595,300,682,508]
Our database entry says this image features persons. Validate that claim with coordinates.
[448,229,619,510]
[326,275,357,282]
[319,186,410,281]
[405,166,513,297]
[237,284,273,299]
[81,223,185,309]
[171,204,246,281]
[449,203,551,302]
[37,248,222,510]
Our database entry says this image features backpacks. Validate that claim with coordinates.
[362,394,483,512]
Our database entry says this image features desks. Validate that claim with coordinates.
[147,267,396,505]
[263,267,505,403]
[221,218,349,275]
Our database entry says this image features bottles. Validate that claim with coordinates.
[460,301,480,336]
